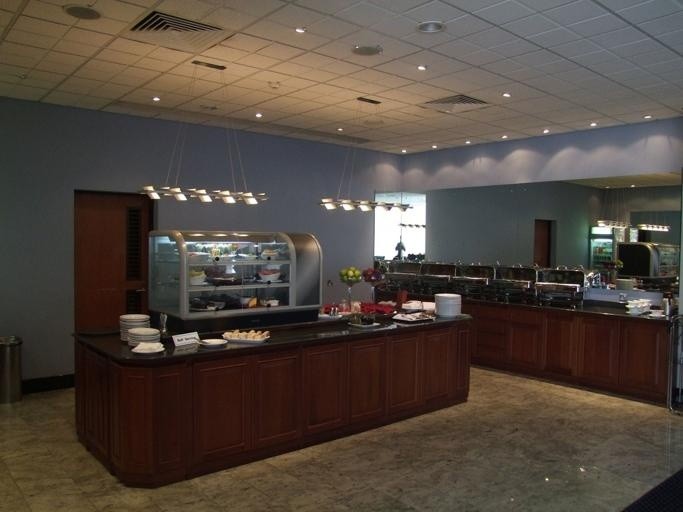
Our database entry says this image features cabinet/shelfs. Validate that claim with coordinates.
[148,229,328,337]
[610,240,678,287]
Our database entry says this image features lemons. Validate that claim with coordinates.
[339,267,361,280]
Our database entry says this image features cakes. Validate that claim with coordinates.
[262,250,280,260]
[258,269,282,281]
[188,250,210,265]
[189,270,207,285]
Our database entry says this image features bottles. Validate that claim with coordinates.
[591,240,612,278]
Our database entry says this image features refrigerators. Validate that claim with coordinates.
[587,224,638,284]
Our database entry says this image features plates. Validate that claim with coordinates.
[224,335,271,345]
[117,313,166,355]
[195,338,227,347]
[434,292,462,319]
[188,249,257,259]
[391,311,433,322]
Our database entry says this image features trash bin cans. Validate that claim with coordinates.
[0,335,22,404]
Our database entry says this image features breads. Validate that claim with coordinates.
[223,329,270,339]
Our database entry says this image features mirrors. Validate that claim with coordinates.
[372,168,682,296]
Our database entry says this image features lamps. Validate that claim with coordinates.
[141,60,272,205]
[318,96,414,214]
[637,180,674,231]
[597,176,634,228]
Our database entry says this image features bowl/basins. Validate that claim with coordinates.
[238,295,255,305]
[258,270,280,281]
[189,274,204,284]
